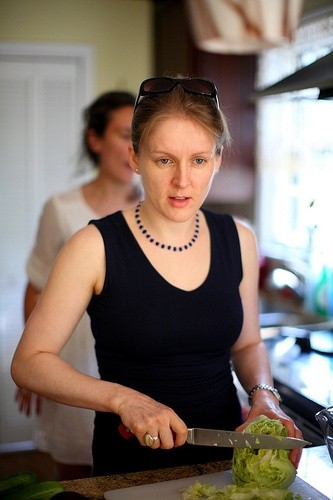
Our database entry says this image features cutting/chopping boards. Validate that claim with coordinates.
[103,466,328,500]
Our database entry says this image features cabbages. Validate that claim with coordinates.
[178,415,305,500]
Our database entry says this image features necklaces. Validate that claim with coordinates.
[134,201,201,251]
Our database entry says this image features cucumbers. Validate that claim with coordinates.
[0,468,65,500]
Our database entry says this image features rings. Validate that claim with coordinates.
[22,389,28,393]
[144,434,159,446]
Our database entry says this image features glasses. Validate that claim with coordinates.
[132,77,220,113]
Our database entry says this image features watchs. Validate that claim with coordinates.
[247,386,282,408]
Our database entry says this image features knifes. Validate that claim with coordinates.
[119,422,312,452]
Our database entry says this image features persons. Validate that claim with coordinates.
[11,72,306,479]
[11,89,143,485]
